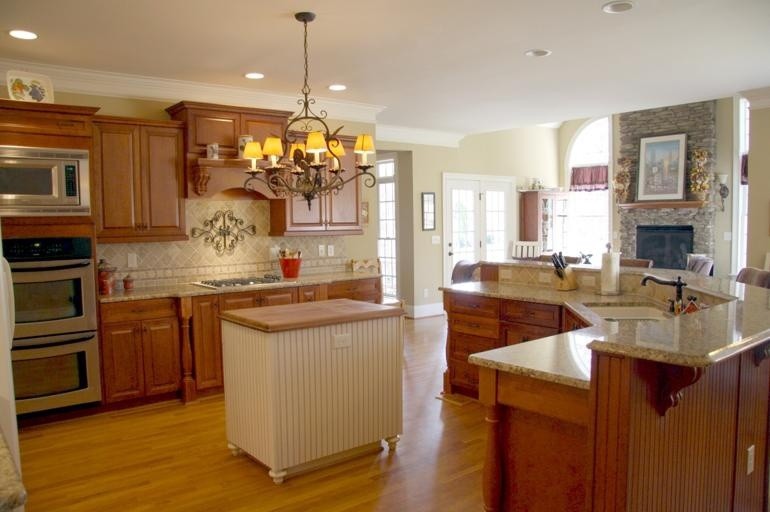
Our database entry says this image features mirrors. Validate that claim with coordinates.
[422,193,435,230]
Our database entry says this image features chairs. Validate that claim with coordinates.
[620,258,653,269]
[684,256,714,276]
[736,267,770,289]
[535,255,582,264]
[512,240,541,260]
[352,258,404,307]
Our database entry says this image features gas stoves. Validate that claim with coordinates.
[191,274,297,290]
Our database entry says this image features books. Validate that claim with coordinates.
[685,254,713,277]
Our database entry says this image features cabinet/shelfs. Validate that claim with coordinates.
[327,278,381,305]
[192,295,222,400]
[164,101,295,158]
[562,307,589,333]
[298,284,328,304]
[267,131,364,237]
[219,287,299,393]
[444,291,562,404]
[517,191,569,256]
[96,297,183,411]
[93,114,189,244]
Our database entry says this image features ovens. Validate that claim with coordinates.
[11,257,103,420]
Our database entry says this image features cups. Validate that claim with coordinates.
[278,258,302,277]
[207,143,220,160]
[238,134,253,159]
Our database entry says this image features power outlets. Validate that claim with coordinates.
[747,445,754,476]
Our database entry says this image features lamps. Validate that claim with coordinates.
[244,12,376,211]
[718,175,729,212]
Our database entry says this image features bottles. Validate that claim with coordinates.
[111,268,122,290]
[97,259,111,296]
[122,274,134,290]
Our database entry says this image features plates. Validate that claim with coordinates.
[6,70,56,103]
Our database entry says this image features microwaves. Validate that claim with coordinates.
[1,157,81,206]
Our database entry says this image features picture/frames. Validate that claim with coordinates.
[634,133,688,202]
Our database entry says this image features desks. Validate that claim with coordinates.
[218,298,406,487]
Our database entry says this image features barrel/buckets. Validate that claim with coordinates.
[279,257,301,278]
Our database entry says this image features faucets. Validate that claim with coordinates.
[642,274,677,289]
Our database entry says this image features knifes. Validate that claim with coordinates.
[551,251,567,276]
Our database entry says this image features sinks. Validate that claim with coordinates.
[583,300,668,323]
